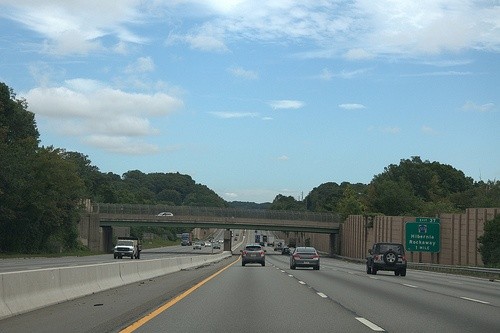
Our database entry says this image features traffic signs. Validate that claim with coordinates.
[405,221,441,252]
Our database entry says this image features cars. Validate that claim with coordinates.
[156,211,174,216]
[239,244,267,266]
[267,239,295,256]
[181,233,223,249]
[288,246,320,270]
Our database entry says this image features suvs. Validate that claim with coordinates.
[366,242,407,277]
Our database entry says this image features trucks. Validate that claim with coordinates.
[113,236,143,260]
